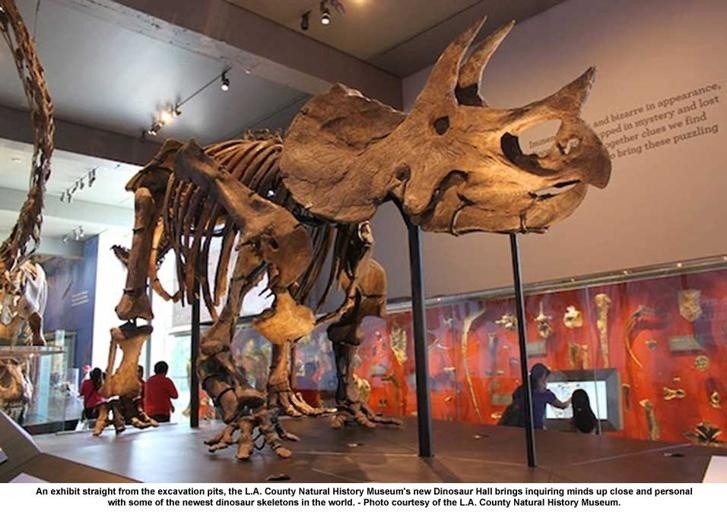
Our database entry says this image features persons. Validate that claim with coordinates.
[79,361,178,428]
[498,363,571,431]
[570,389,598,435]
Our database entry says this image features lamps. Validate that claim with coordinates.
[147,125,162,135]
[219,70,230,92]
[299,2,332,33]
